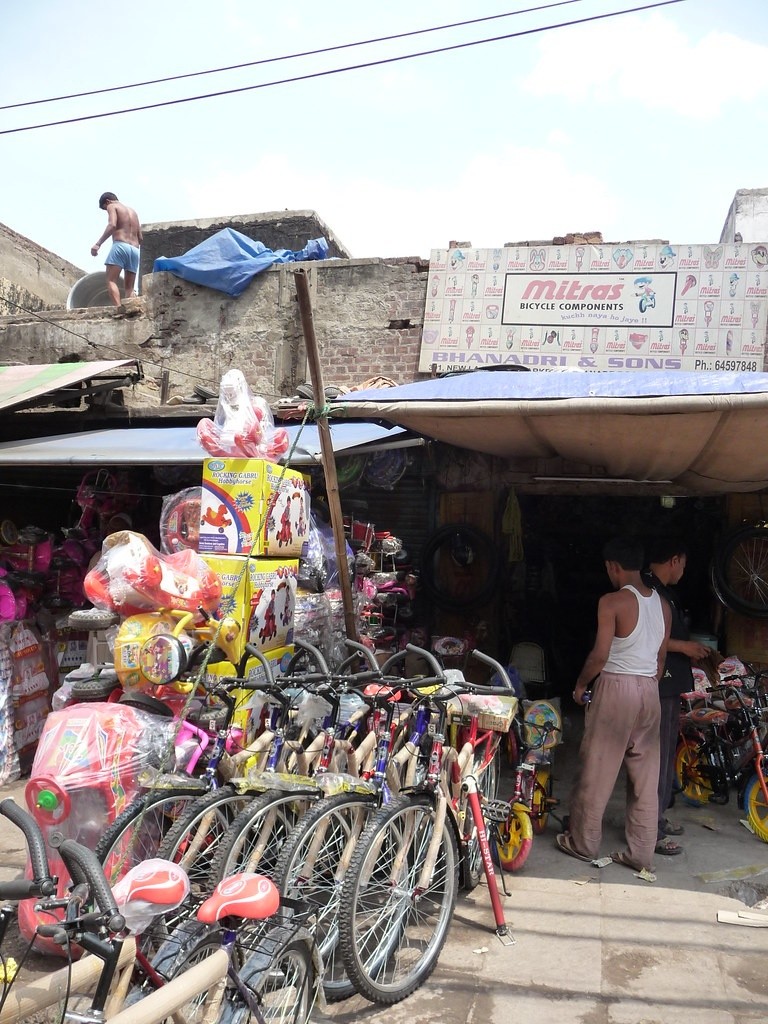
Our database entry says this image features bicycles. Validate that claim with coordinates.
[706,512,768,620]
[0,468,132,622]
[62,538,245,699]
[673,653,768,843]
[0,797,241,1024]
[489,700,571,872]
[39,837,323,1024]
[76,639,515,1003]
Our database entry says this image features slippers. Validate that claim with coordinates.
[654,836,682,855]
[556,833,594,862]
[606,851,656,872]
[660,821,684,835]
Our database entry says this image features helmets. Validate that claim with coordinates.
[450,545,473,569]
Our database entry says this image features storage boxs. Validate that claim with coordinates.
[53,637,88,689]
[177,457,311,758]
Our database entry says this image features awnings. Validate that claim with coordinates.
[0,421,418,464]
[324,368,768,495]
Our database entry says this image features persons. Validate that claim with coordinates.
[91,193,144,305]
[557,538,711,874]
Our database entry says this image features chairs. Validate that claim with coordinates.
[507,639,554,700]
